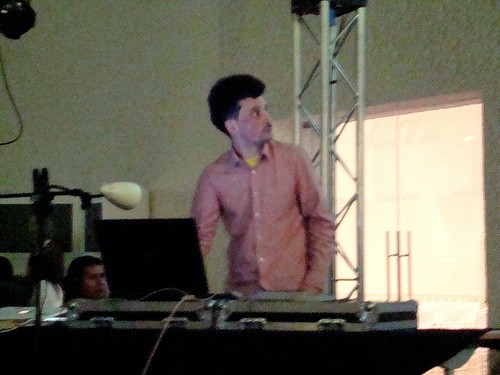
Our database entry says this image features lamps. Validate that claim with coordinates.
[0,0,37,40]
[99,181,142,210]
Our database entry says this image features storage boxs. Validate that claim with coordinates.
[68,294,419,331]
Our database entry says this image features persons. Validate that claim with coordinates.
[191,73,336,295]
[0,242,110,314]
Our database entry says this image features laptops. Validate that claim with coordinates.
[0,306,68,326]
[94,219,232,300]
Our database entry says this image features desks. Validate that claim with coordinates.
[0,315,500,375]
